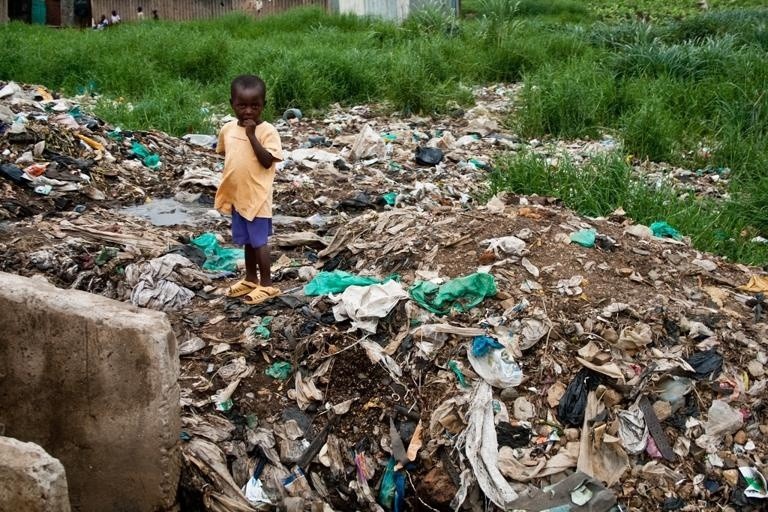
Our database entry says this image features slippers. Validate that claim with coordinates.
[244,285,281,304]
[224,278,259,298]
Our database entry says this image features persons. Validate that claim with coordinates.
[152,10,158,20]
[696,0,708,10]
[76,0,120,31]
[136,7,144,20]
[214,75,283,305]
